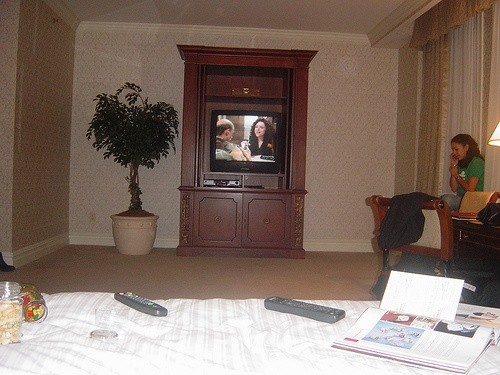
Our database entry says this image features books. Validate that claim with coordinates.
[331,270,500,375]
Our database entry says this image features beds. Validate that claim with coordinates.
[0,292,500,375]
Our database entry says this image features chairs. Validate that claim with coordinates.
[374,195,495,305]
[451,191,500,213]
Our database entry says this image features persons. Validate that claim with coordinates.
[440,134,485,211]
[216,119,249,161]
[248,118,275,156]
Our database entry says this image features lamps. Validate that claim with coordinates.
[487,121,500,147]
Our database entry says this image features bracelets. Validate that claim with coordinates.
[456,175,464,184]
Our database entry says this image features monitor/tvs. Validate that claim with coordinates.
[210,109,286,174]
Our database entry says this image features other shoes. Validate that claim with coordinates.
[0,252,15,272]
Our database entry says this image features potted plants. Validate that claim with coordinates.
[86,82,180,254]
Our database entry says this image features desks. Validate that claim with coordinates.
[453,219,500,256]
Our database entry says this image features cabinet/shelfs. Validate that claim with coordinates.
[175,43,319,259]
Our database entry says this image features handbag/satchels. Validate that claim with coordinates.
[477,203,500,226]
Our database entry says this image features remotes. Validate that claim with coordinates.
[114,291,168,317]
[264,296,345,324]
